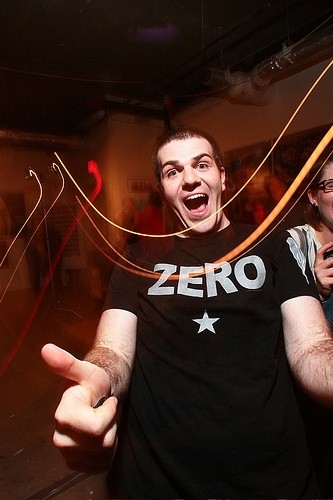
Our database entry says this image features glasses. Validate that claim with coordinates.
[312,179,332,190]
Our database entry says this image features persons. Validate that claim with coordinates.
[41,129,333,500]
[286,142,333,303]
